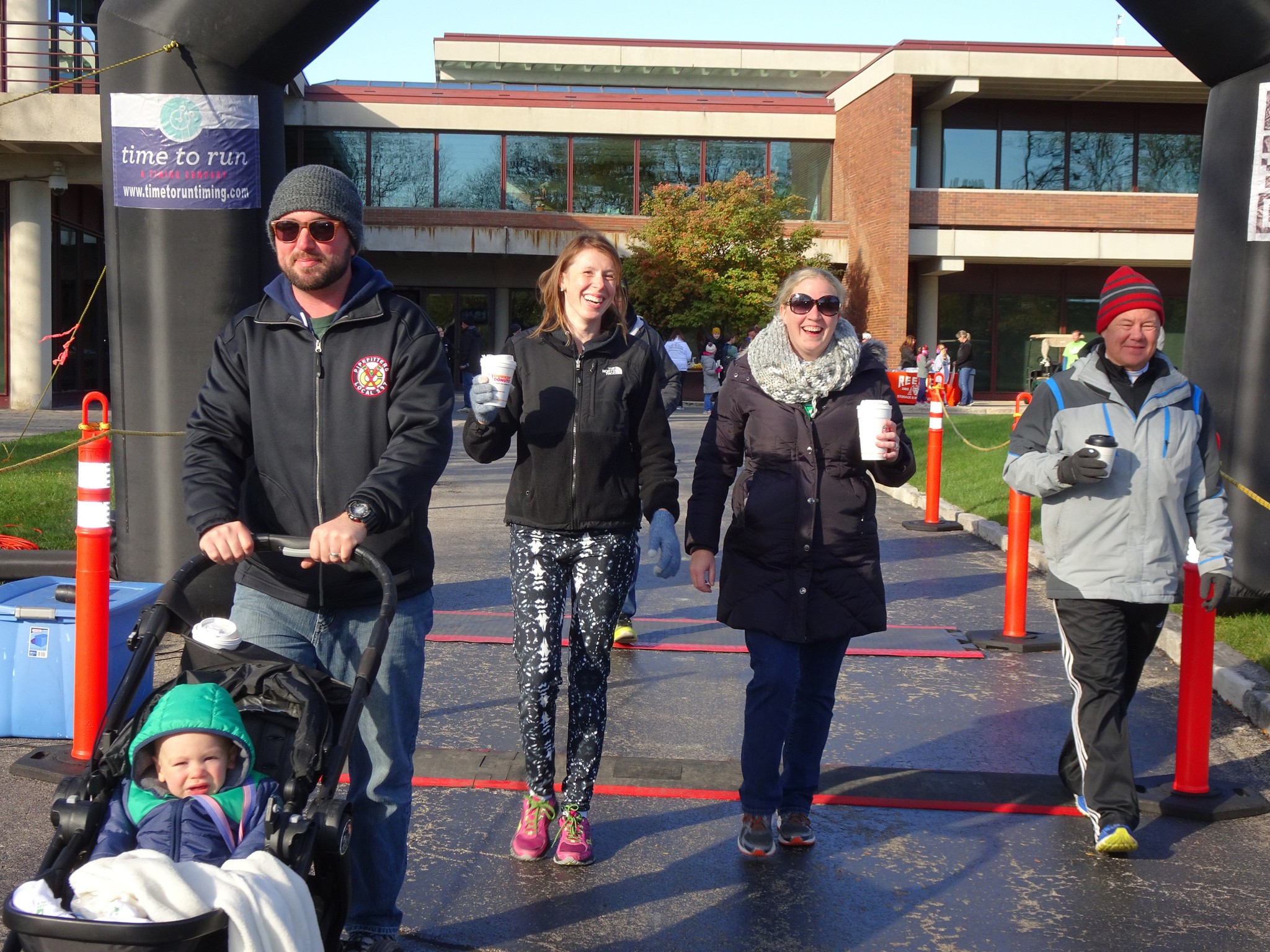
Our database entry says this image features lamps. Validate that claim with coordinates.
[48,160,68,191]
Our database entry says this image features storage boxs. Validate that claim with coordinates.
[0,574,164,741]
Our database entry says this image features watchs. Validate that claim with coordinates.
[342,500,381,536]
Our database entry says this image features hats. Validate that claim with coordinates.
[264,166,364,261]
[712,328,720,335]
[728,345,738,356]
[706,342,717,354]
[1096,265,1166,334]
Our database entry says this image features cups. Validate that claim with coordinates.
[856,400,893,460]
[480,354,517,408]
[1084,435,1119,478]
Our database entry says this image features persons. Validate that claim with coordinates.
[1061,330,1087,371]
[899,335,916,370]
[1003,266,1233,852]
[930,344,951,406]
[859,331,872,344]
[684,266,916,859]
[953,330,976,407]
[915,345,934,407]
[434,309,767,642]
[462,231,681,866]
[88,683,282,871]
[183,164,456,952]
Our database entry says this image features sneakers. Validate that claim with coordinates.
[777,811,815,844]
[509,793,560,862]
[1095,823,1138,852]
[1074,792,1092,819]
[551,803,595,867]
[737,809,775,856]
[613,618,639,644]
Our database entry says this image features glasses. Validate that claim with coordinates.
[784,292,843,318]
[271,219,341,243]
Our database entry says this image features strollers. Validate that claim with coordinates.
[0,533,397,951]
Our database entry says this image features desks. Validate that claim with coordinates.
[885,371,961,406]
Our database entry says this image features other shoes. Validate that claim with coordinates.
[915,401,930,406]
[965,401,974,406]
[676,406,686,410]
[342,932,398,952]
[703,410,712,415]
[956,402,967,407]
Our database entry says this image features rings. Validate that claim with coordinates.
[896,444,899,451]
[330,552,340,558]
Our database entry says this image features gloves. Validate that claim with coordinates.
[1057,447,1109,485]
[648,510,681,579]
[715,366,722,374]
[468,374,514,424]
[1199,572,1232,611]
